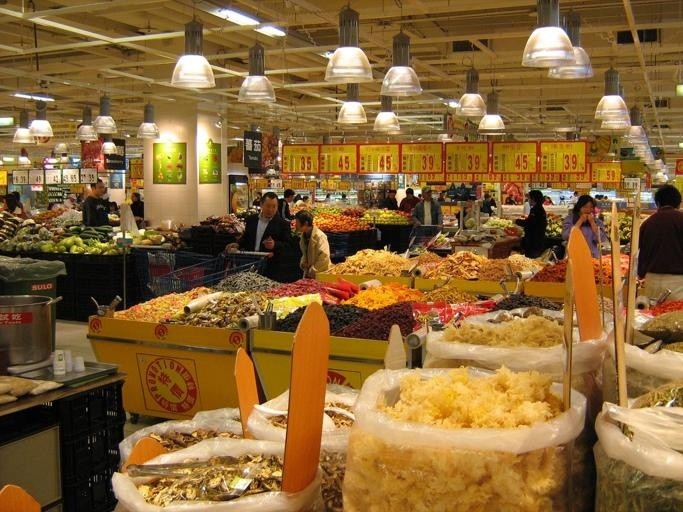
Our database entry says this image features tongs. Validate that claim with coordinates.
[125,461,251,501]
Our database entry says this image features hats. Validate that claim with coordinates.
[422,186,432,192]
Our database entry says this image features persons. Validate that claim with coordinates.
[483,194,515,215]
[562,191,608,257]
[225,188,330,282]
[341,194,347,201]
[64,179,144,227]
[1,192,24,217]
[514,190,553,259]
[380,186,469,234]
[638,184,683,277]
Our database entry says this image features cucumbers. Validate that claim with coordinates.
[79,225,112,240]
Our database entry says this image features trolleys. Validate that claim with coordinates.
[145,251,215,294]
[405,223,443,254]
[152,248,275,301]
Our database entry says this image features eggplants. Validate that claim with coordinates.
[54,235,87,255]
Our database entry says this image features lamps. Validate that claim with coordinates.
[231,46,282,109]
[132,99,161,143]
[98,139,119,159]
[211,4,288,40]
[77,89,117,145]
[321,7,375,86]
[8,88,56,148]
[519,0,595,81]
[444,68,507,138]
[334,87,367,126]
[371,94,402,136]
[591,63,631,132]
[623,106,670,184]
[380,26,424,99]
[166,18,224,95]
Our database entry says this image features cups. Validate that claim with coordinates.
[51,348,85,375]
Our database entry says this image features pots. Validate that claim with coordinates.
[0,295,64,365]
